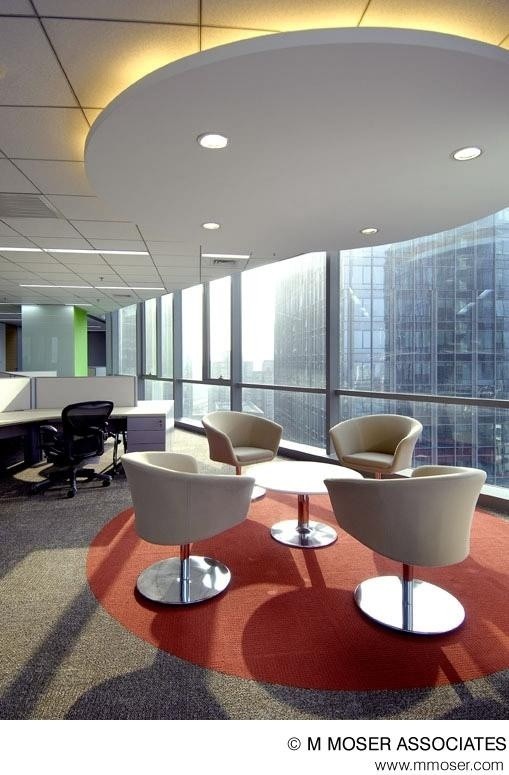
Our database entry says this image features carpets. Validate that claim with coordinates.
[84,484,509,690]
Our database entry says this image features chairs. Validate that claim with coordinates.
[330,413,423,480]
[202,411,283,503]
[33,401,113,498]
[324,462,487,635]
[122,450,257,608]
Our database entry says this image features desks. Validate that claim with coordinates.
[0,400,174,452]
[243,461,364,552]
